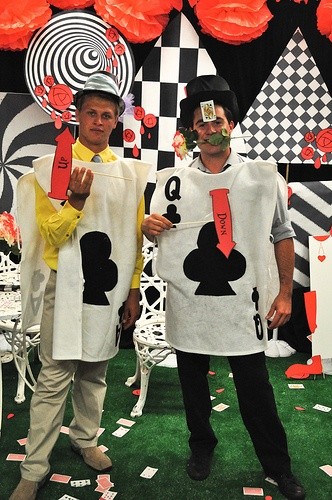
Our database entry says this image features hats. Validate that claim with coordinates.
[74,70,126,118]
[180,74,240,130]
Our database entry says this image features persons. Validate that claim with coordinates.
[140,75,307,500]
[9,70,152,500]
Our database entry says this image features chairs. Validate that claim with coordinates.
[0,251,42,405]
[123,240,178,418]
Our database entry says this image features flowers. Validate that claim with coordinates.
[172,126,252,161]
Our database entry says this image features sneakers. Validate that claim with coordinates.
[264,470,306,500]
[187,447,212,481]
[9,475,47,500]
[71,442,113,474]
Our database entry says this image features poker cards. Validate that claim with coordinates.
[5,378,332,499]
[1,1,332,500]
